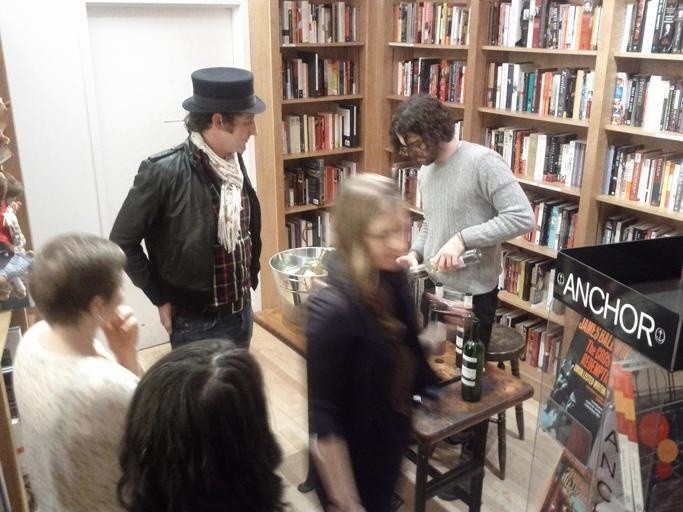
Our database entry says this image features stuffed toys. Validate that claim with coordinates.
[0,168,34,302]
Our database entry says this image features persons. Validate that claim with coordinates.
[391,95,537,501]
[108,66,263,358]
[308,172,446,511]
[115,338,317,512]
[10,231,146,511]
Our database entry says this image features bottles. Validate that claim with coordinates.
[405,248,482,279]
[456,291,486,403]
[424,282,474,354]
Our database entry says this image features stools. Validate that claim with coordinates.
[457,315,525,477]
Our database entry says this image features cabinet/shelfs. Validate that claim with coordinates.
[249,0,683,397]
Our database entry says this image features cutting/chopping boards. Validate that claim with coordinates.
[426,341,462,386]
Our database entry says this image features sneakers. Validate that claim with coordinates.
[438,480,472,501]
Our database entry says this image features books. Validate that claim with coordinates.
[482,2,603,372]
[281,1,361,265]
[537,315,683,512]
[593,1,683,245]
[390,3,470,318]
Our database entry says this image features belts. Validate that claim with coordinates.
[200,292,252,318]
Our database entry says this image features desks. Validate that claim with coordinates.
[258,296,535,509]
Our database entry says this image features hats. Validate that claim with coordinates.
[181,66,267,114]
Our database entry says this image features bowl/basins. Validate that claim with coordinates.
[267,245,332,319]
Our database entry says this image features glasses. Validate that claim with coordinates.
[401,141,424,155]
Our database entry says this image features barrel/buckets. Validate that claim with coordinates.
[268,247,337,327]
